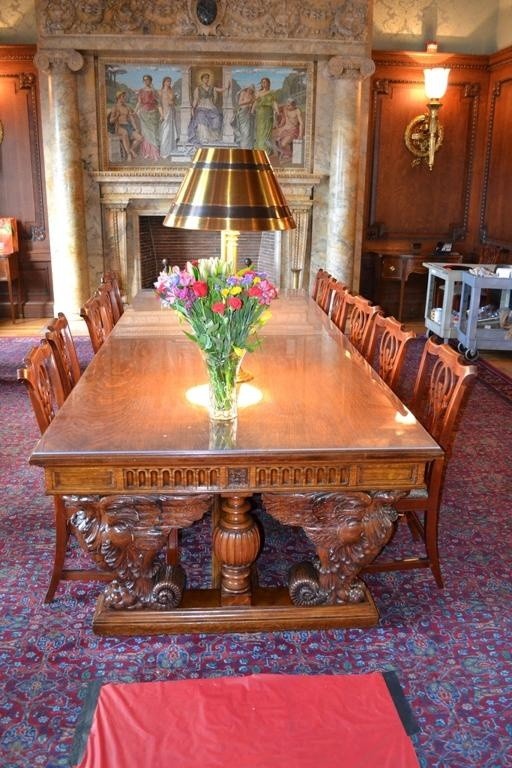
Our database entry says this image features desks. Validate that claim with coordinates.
[368,248,464,321]
[29,287,444,638]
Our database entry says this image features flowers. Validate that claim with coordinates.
[154,255,278,407]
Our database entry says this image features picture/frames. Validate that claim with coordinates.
[95,53,314,173]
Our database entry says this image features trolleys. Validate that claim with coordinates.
[420,262,512,350]
[457,267,512,360]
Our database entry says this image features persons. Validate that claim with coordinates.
[107,68,305,165]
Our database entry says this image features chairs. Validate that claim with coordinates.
[436,243,505,313]
[311,269,417,394]
[357,334,479,590]
[47,309,84,400]
[15,341,188,602]
[78,269,124,354]
[18,338,66,435]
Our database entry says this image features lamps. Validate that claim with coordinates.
[163,142,294,384]
[404,62,452,177]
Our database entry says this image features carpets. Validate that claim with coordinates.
[0,334,512,768]
